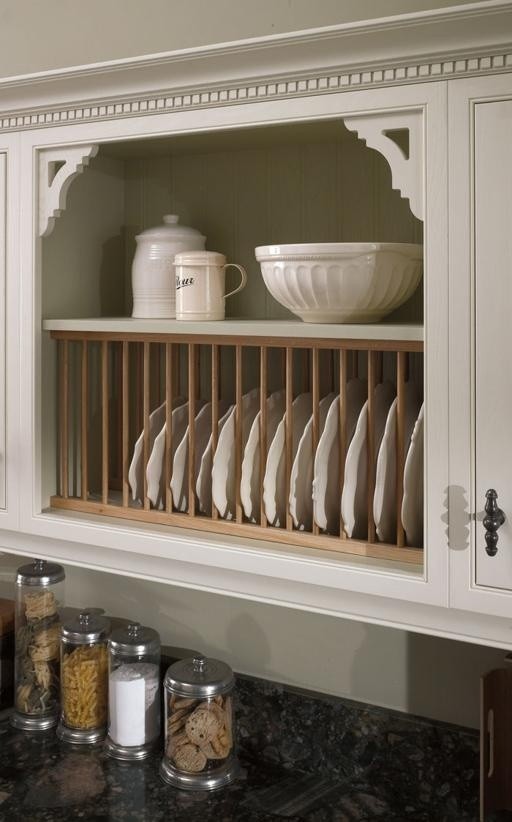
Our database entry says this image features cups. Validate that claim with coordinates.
[171,250,247,323]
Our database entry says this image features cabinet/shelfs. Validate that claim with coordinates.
[0,0,512,651]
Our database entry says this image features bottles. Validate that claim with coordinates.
[103,622,162,762]
[160,656,240,793]
[55,612,111,746]
[11,558,66,732]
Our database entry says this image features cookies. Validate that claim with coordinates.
[168,694,233,773]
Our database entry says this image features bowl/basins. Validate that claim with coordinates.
[253,241,423,326]
